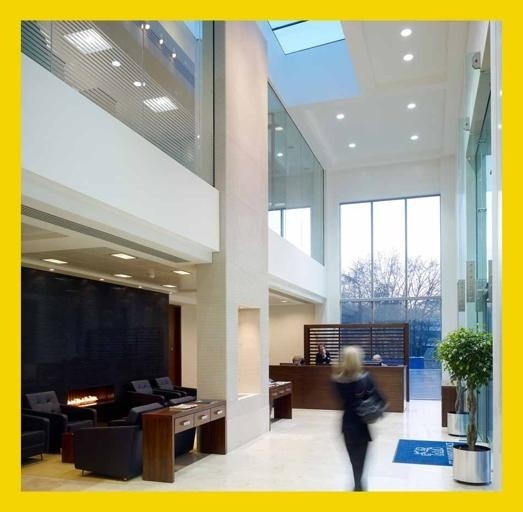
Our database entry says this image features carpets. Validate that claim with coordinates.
[392,439,464,466]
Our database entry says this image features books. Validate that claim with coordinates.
[193,399,215,404]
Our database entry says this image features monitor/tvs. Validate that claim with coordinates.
[362,361,381,367]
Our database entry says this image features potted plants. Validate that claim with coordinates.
[436,326,497,488]
[443,359,472,436]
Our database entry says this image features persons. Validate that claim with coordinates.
[372,354,388,367]
[330,345,387,491]
[292,356,301,365]
[315,343,331,364]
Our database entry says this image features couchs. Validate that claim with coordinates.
[21,408,47,468]
[122,396,197,459]
[70,396,165,482]
[123,376,182,407]
[154,373,198,401]
[22,385,98,453]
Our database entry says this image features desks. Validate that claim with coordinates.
[268,380,294,432]
[137,398,228,485]
